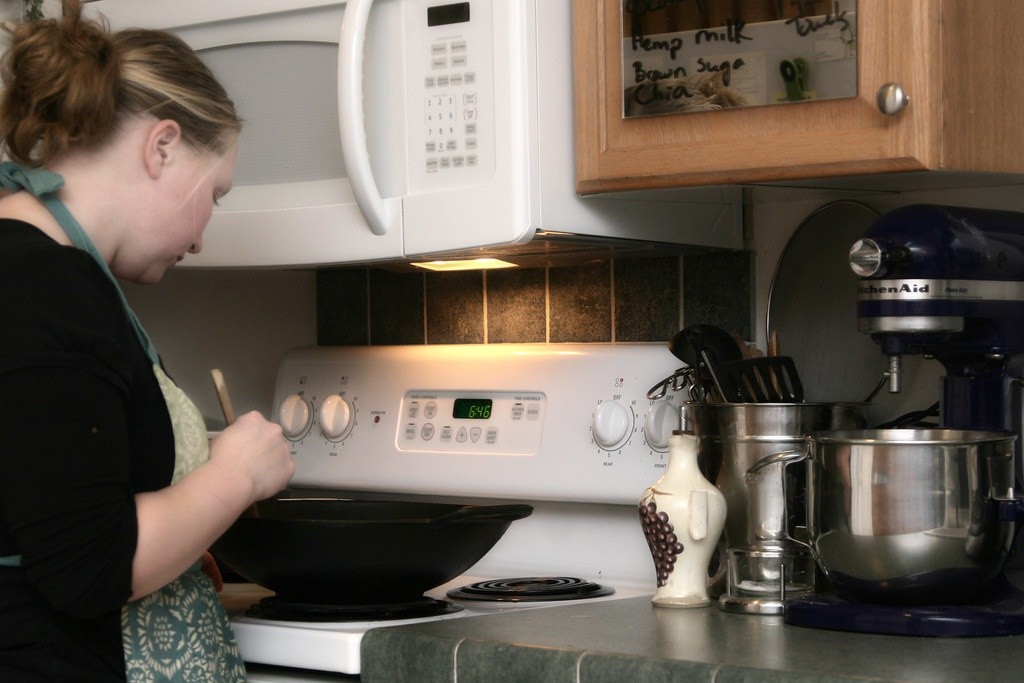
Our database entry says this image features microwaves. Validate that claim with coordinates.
[75,1,747,274]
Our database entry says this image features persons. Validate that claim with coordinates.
[0,0,295,683]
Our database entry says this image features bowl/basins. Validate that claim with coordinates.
[806,427,1018,603]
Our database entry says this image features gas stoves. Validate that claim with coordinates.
[215,343,752,677]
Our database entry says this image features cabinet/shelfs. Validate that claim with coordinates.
[571,0,1024,195]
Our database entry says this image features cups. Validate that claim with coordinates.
[714,402,868,611]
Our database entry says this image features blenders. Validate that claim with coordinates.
[783,205,1024,639]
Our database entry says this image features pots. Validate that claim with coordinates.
[198,499,537,606]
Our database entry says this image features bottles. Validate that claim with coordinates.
[637,406,726,605]
